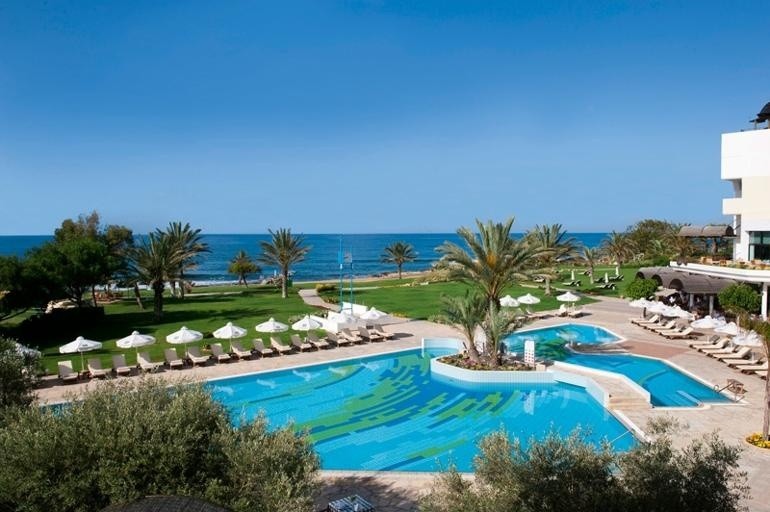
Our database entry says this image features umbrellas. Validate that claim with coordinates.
[362,362,387,371]
[116,330,156,356]
[361,308,388,330]
[332,311,359,332]
[58,335,101,371]
[327,367,356,377]
[627,296,766,351]
[167,325,203,355]
[14,343,42,378]
[499,294,519,308]
[257,378,285,390]
[218,383,242,394]
[293,371,319,381]
[213,321,246,350]
[556,291,582,310]
[292,317,321,336]
[255,316,289,337]
[518,293,540,310]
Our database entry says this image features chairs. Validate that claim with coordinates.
[593,274,624,289]
[562,280,581,286]
[701,256,766,270]
[578,271,588,275]
[514,303,583,324]
[534,279,552,283]
[629,312,769,380]
